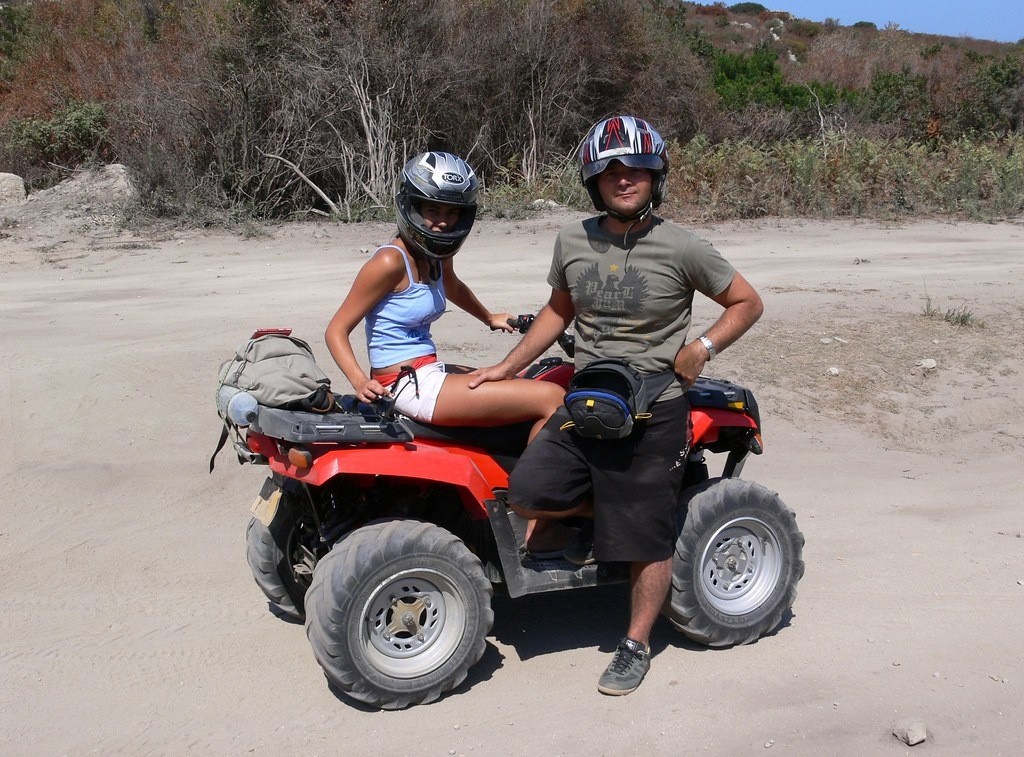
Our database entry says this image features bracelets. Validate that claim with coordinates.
[697,334,716,362]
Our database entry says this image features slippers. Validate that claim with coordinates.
[526,527,589,558]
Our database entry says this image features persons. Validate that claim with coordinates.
[324,151,584,560]
[468,115,764,696]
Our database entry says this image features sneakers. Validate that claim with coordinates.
[597,637,651,695]
[564,531,599,565]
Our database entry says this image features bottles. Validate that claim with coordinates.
[228,391,259,426]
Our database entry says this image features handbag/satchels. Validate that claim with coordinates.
[559,358,679,440]
[218,333,334,412]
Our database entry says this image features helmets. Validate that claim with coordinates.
[579,116,671,211]
[395,151,478,259]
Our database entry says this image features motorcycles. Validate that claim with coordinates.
[209,313,806,710]
